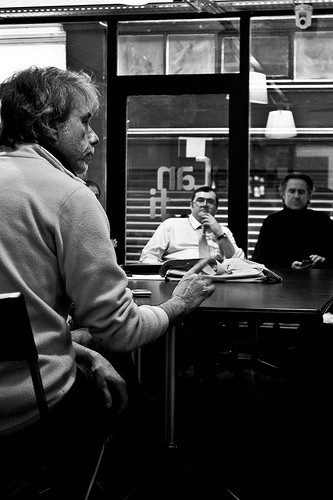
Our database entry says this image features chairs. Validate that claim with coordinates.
[0,290,143,500]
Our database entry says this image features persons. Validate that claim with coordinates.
[139,185,249,383]
[247,173,333,381]
[1,64,217,500]
[82,177,118,248]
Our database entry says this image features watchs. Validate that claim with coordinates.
[217,233,226,240]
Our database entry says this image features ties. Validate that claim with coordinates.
[199,225,210,257]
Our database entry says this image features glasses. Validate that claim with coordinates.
[193,199,217,207]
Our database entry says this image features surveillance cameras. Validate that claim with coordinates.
[295,4,314,29]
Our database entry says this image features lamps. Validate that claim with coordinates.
[265,108,296,140]
[226,67,268,104]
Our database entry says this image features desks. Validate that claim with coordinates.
[124,273,332,451]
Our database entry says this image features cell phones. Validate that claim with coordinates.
[301,260,313,266]
[131,288,152,298]
